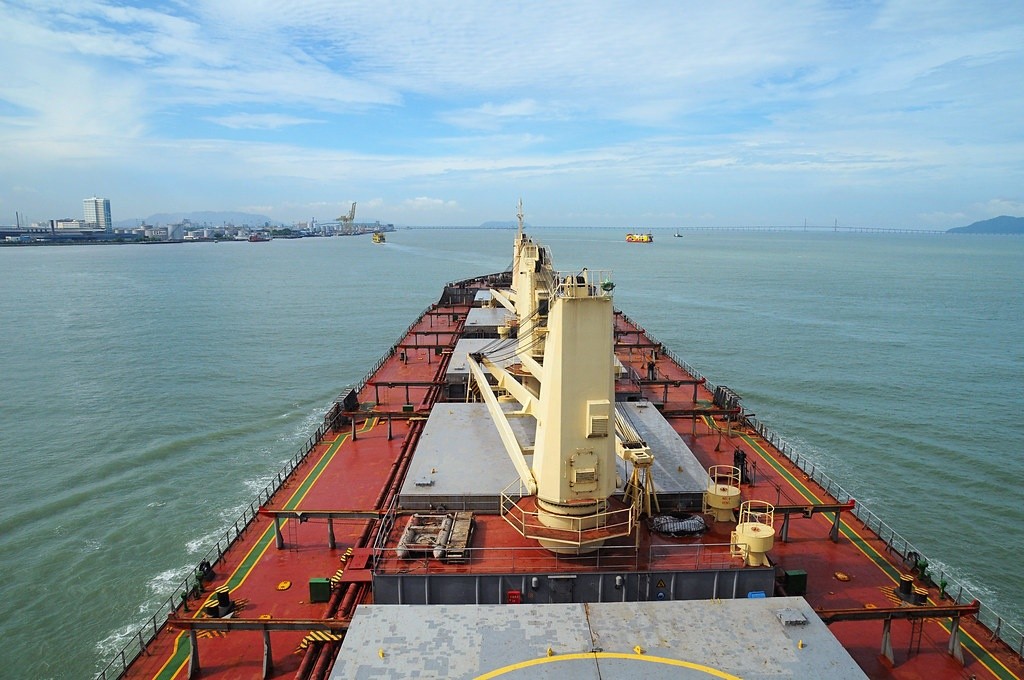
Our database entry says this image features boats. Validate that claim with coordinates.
[625,229,655,243]
[372,227,386,245]
